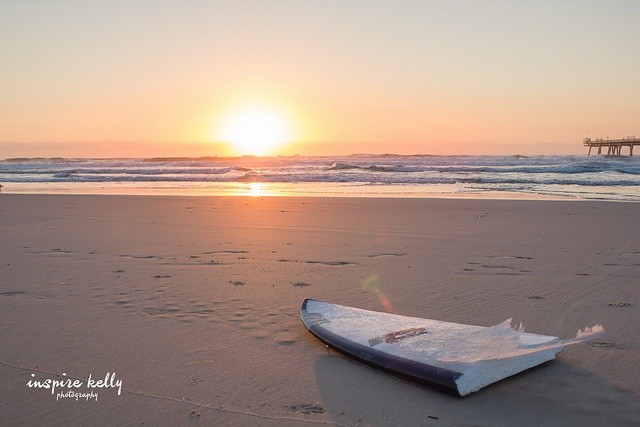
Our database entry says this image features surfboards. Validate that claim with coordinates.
[293,294,606,402]
